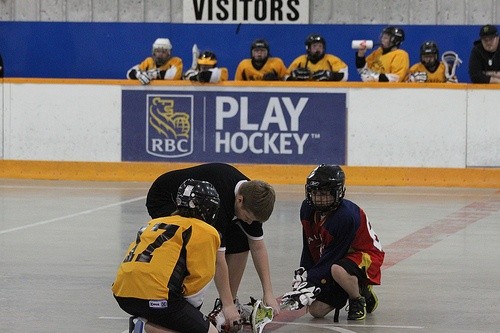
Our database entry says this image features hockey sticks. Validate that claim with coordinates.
[249,299,295,333]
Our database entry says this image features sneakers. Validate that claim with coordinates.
[346,294,366,320]
[231,296,253,322]
[362,284,378,313]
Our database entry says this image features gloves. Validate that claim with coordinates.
[291,68,310,80]
[410,72,426,82]
[313,69,331,81]
[147,70,162,79]
[291,267,308,290]
[283,286,317,310]
[361,69,380,82]
[136,71,153,85]
[196,69,212,83]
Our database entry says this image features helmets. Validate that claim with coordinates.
[305,163,345,212]
[251,40,269,63]
[383,26,404,45]
[197,52,217,71]
[420,41,438,66]
[177,179,219,224]
[153,38,172,63]
[305,35,325,61]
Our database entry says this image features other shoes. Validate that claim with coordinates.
[128,317,146,333]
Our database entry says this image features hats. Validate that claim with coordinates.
[479,25,497,37]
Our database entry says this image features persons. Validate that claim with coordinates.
[184,52,228,83]
[145,163,280,333]
[111,179,220,333]
[403,40,457,83]
[280,164,385,321]
[126,38,184,85]
[284,35,349,81]
[356,26,409,82]
[469,25,500,84]
[235,39,287,81]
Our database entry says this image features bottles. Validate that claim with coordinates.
[351,40,381,49]
